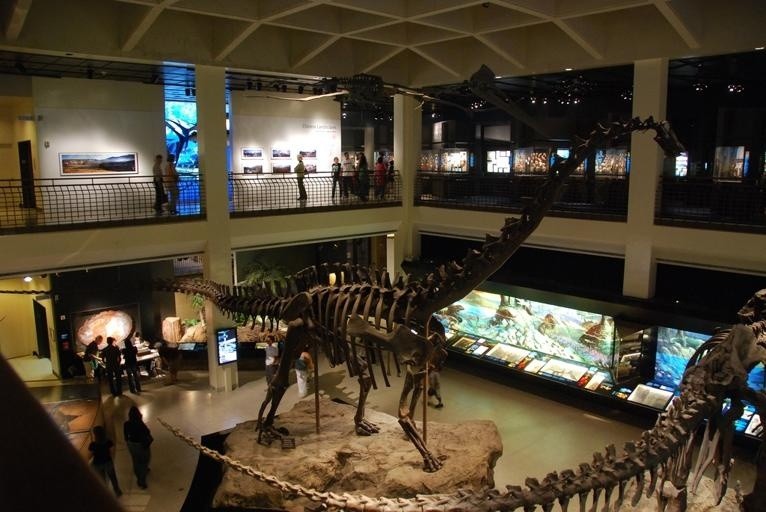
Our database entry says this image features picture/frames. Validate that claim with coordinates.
[299,145,317,159]
[271,145,293,160]
[58,152,138,178]
[241,162,263,174]
[242,148,263,159]
[304,164,316,172]
[173,256,203,276]
[271,161,291,173]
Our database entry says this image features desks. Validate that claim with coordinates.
[96,348,160,382]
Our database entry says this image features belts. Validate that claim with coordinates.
[127,442,145,446]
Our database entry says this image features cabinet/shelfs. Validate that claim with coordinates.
[434,326,766,463]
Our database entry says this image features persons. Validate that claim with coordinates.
[123,406,155,490]
[153,155,165,213]
[375,157,387,200]
[293,155,309,201]
[81,334,103,385]
[294,350,314,398]
[341,153,355,200]
[100,337,123,397]
[332,157,342,199]
[263,335,278,391]
[122,338,141,394]
[88,425,123,498]
[165,154,181,218]
[425,318,448,407]
[359,155,371,200]
